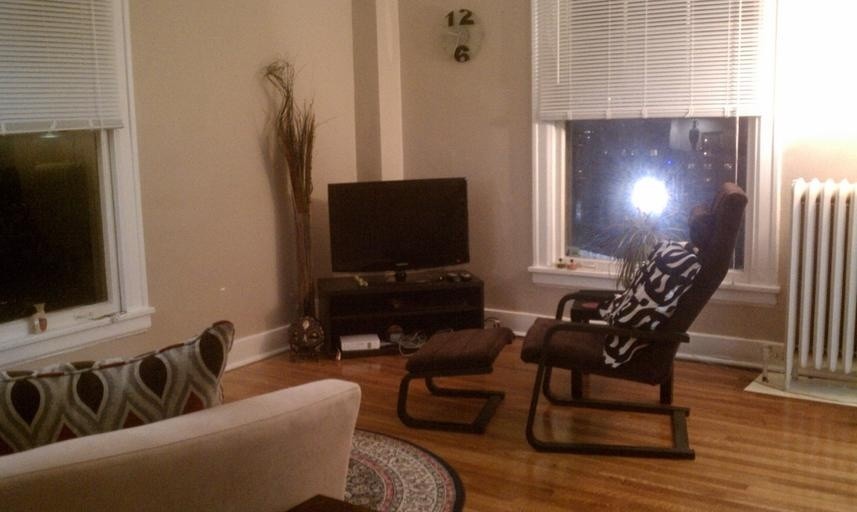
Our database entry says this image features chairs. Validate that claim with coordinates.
[521,181,747,459]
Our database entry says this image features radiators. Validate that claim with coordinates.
[786,178,856,385]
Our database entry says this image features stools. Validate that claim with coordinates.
[398,328,514,433]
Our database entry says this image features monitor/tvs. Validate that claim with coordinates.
[327,177,470,282]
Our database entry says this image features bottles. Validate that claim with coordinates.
[31,303,47,333]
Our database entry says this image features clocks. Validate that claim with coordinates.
[432,9,484,64]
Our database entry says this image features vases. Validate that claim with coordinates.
[288,304,324,360]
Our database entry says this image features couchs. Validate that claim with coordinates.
[1,379,363,511]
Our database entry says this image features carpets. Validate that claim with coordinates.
[743,370,857,409]
[346,428,465,512]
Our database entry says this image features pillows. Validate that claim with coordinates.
[597,238,701,369]
[0,318,234,458]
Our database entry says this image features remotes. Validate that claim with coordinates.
[445,272,460,284]
[460,271,472,281]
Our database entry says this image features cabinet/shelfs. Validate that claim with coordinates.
[317,268,484,356]
